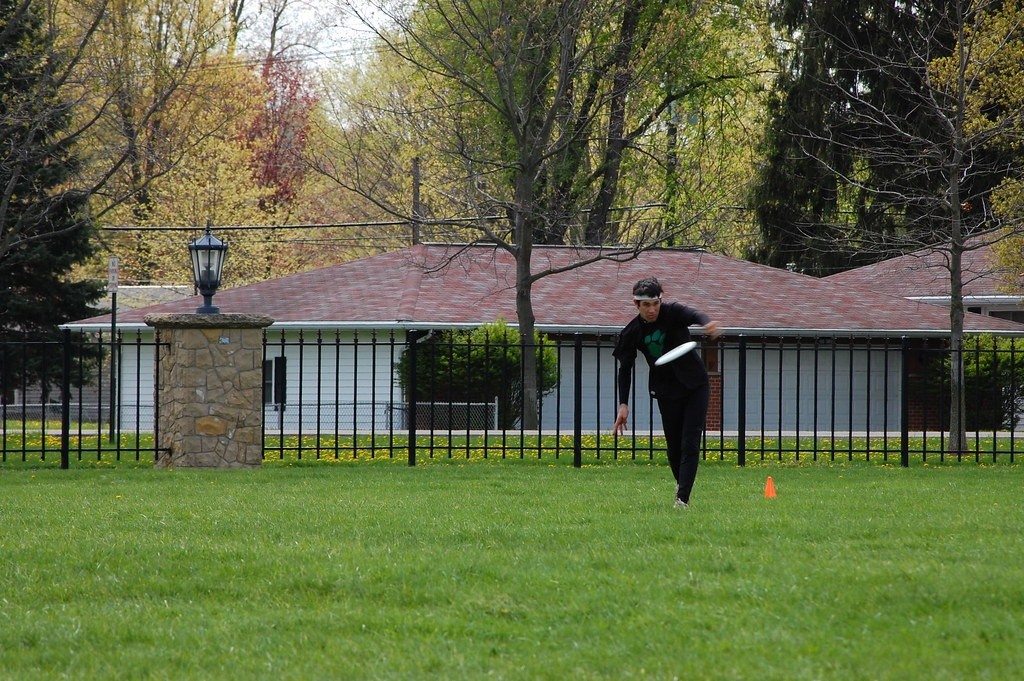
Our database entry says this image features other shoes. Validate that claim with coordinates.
[674,484,688,510]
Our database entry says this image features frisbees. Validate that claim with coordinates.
[654,340,698,367]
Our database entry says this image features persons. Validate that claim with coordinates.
[612,277,719,513]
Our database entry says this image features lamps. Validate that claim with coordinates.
[188,217,229,314]
